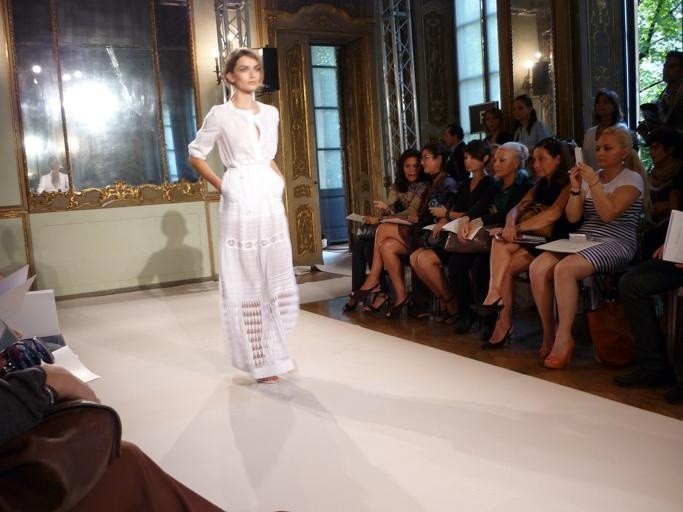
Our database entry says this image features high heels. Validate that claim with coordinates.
[537,340,552,357]
[360,292,391,314]
[470,296,506,319]
[544,337,576,369]
[481,322,515,349]
[385,291,415,319]
[343,296,367,312]
[455,310,482,334]
[349,282,384,299]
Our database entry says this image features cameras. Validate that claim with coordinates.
[430,198,439,207]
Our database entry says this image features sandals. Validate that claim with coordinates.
[434,295,447,323]
[445,293,464,324]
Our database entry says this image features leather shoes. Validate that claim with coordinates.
[664,390,681,402]
[613,364,676,387]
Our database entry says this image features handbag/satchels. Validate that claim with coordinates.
[357,223,378,239]
[518,200,558,240]
[409,225,446,250]
[586,282,653,369]
[444,225,493,253]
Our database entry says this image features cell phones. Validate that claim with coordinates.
[575,147,583,167]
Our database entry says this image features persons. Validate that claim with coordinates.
[36,155,69,194]
[0,271,222,512]
[1,270,222,510]
[187,48,299,384]
[343,49,683,405]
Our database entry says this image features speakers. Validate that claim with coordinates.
[251,47,280,91]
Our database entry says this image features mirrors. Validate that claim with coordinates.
[7,1,211,212]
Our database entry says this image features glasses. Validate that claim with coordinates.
[421,154,434,160]
